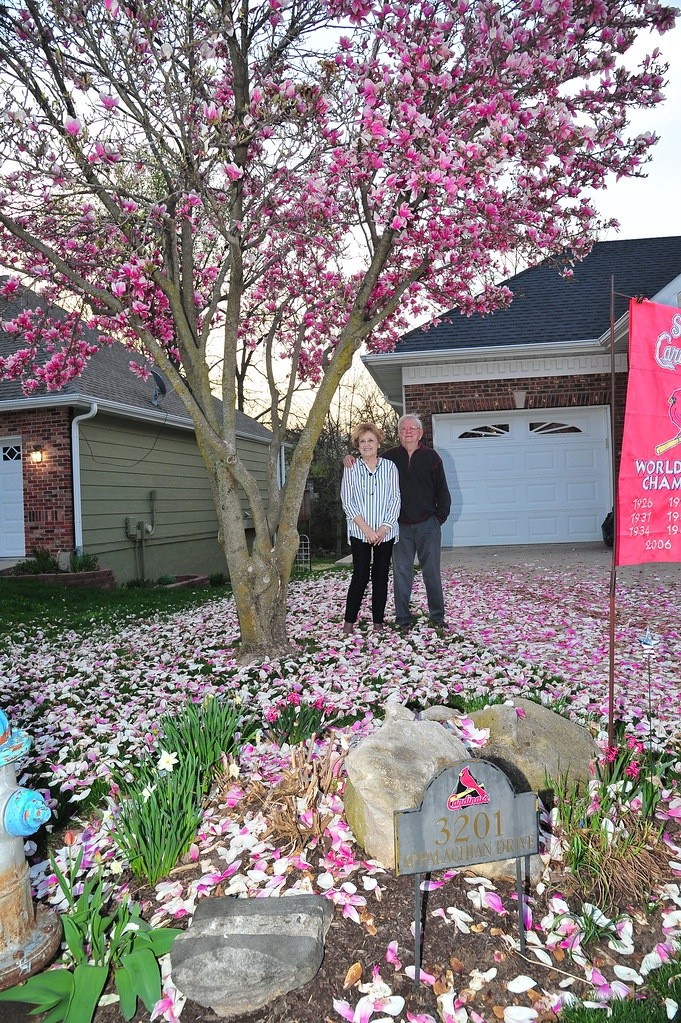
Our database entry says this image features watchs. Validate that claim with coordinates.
[383,525,390,533]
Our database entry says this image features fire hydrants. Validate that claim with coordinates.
[0,708,63,991]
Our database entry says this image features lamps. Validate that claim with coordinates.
[31,445,42,464]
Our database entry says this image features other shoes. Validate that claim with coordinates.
[374,623,383,630]
[429,621,449,628]
[400,624,411,633]
[343,621,353,639]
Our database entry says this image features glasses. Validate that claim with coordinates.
[400,425,420,432]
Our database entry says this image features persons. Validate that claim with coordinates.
[343,414,452,637]
